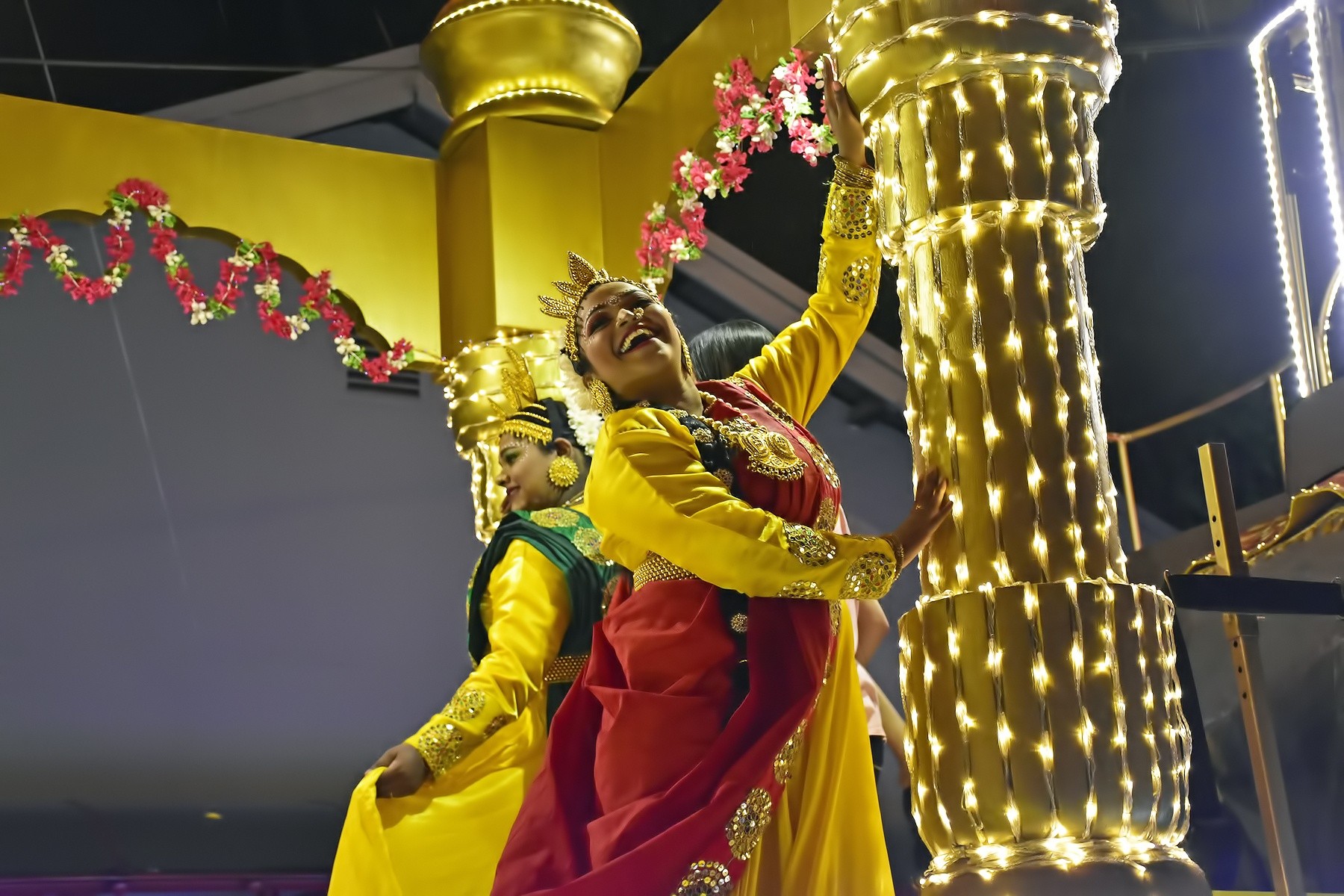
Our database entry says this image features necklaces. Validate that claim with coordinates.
[670,390,795,463]
[562,491,584,506]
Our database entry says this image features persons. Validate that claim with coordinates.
[328,396,623,896]
[684,319,889,783]
[489,57,955,896]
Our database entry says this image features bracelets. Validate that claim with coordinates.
[831,156,875,190]
[879,533,904,582]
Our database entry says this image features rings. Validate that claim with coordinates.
[916,504,922,510]
[390,792,394,798]
